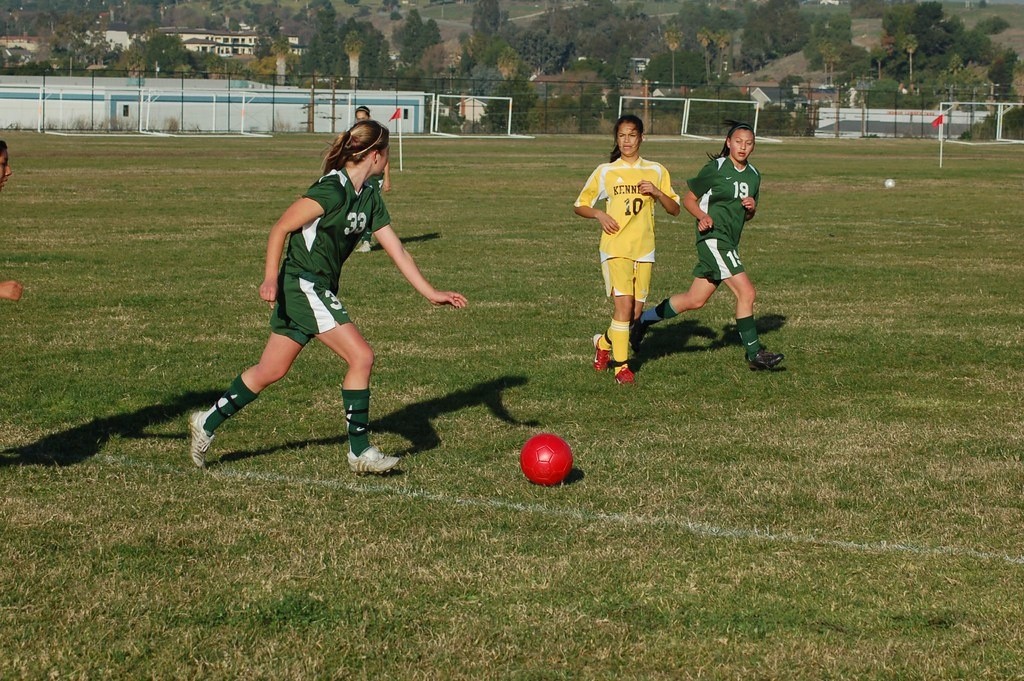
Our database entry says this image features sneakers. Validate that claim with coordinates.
[346,445,400,474]
[355,241,372,252]
[629,310,651,353]
[614,367,634,385]
[592,333,611,372]
[187,411,215,467]
[745,348,784,371]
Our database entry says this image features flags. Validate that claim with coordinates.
[931,113,944,128]
[387,107,402,122]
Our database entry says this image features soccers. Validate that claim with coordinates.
[884,178,896,189]
[519,434,574,487]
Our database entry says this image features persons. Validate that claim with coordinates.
[628,122,785,373]
[0,137,24,302]
[354,105,392,253]
[573,113,681,388]
[187,119,469,474]
